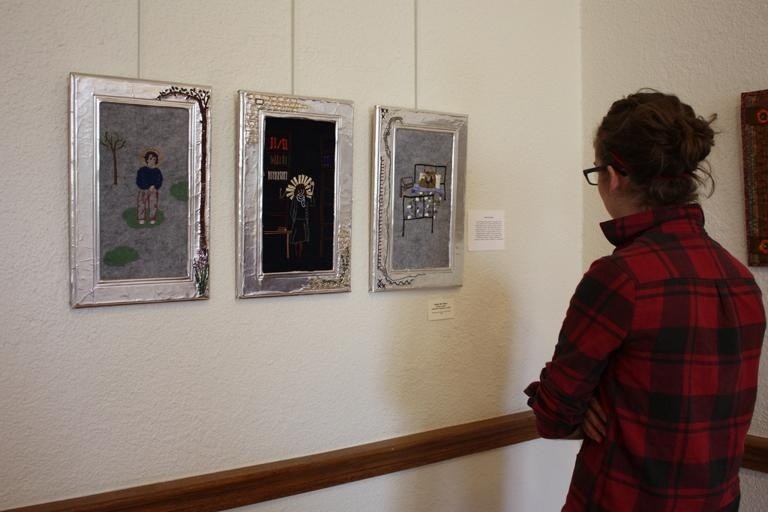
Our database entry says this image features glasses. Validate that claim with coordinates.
[583,164,628,186]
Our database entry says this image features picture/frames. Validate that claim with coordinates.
[236,89,356,301]
[67,70,210,308]
[368,105,470,293]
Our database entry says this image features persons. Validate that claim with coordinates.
[524,85,767,512]
